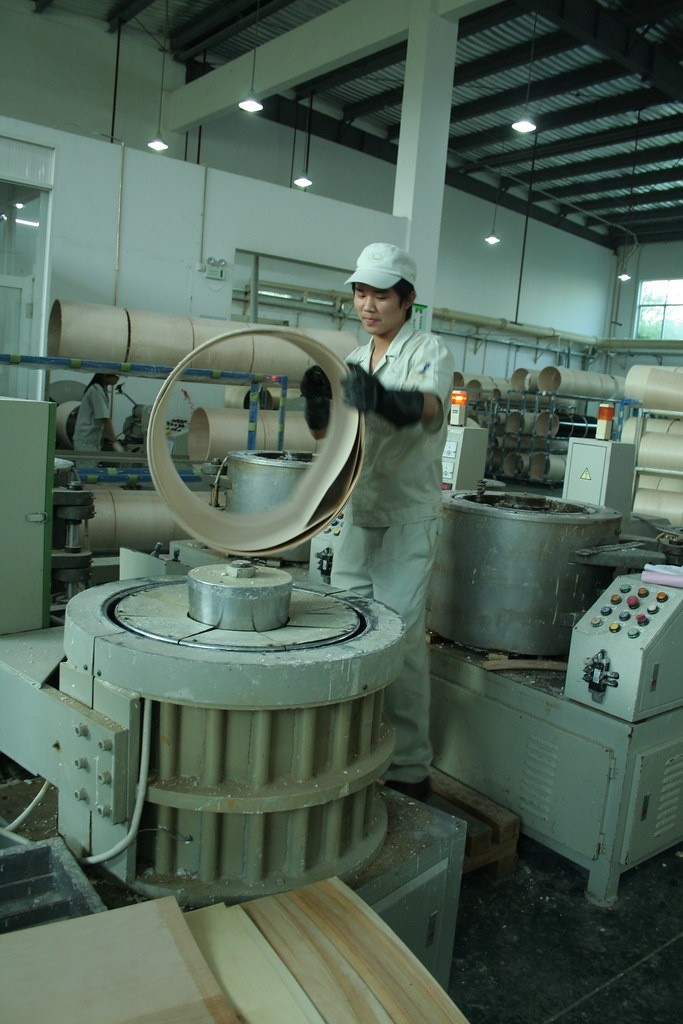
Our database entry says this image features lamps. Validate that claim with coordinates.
[238,0,264,112]
[293,92,313,188]
[484,136,500,245]
[511,2,536,134]
[148,0,168,152]
[206,257,226,267]
[617,101,631,281]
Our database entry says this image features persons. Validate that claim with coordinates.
[310,243,454,793]
[73,373,121,453]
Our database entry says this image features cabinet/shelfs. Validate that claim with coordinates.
[454,387,643,488]
[0,352,288,483]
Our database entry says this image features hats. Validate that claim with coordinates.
[114,374,123,377]
[343,243,416,290]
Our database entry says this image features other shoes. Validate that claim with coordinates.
[383,774,433,803]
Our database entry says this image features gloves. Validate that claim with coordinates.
[340,364,425,426]
[299,365,331,430]
[112,441,123,453]
